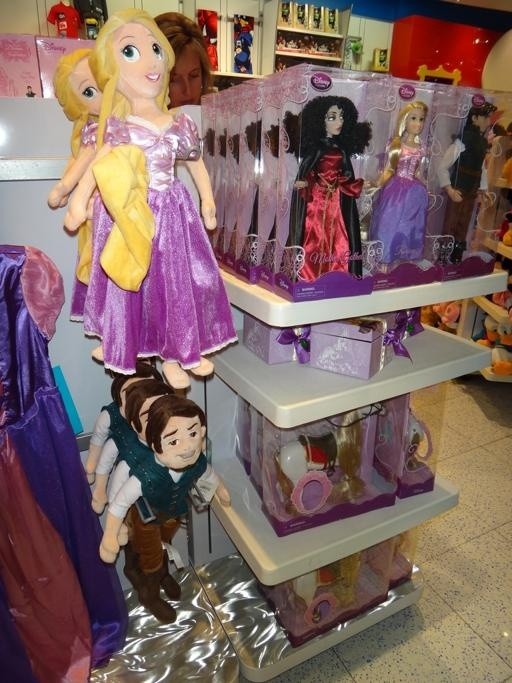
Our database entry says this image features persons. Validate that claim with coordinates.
[366,101,432,273]
[284,95,372,282]
[435,102,496,266]
[47,49,110,362]
[154,12,212,109]
[83,364,231,621]
[65,10,239,389]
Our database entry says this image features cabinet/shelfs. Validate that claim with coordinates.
[260,0,356,74]
[180,244,512,683]
[420,137,511,394]
[0,0,263,81]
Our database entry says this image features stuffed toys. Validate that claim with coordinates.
[431,158,511,375]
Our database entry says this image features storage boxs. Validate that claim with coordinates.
[240,309,311,367]
[34,35,98,98]
[310,301,422,379]
[0,33,44,98]
